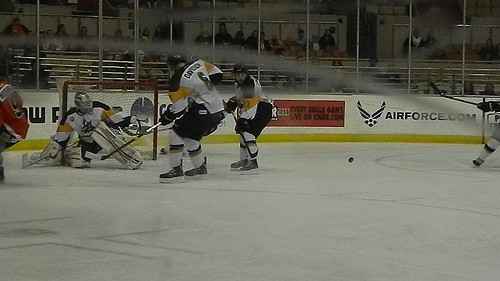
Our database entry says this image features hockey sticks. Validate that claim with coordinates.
[100,121,163,160]
[21,152,53,169]
[230,110,260,160]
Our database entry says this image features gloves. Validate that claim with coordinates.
[224,97,238,113]
[234,117,251,133]
[159,104,177,125]
[477,100,499,112]
[39,138,62,159]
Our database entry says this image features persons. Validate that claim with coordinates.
[472,99,500,166]
[30,91,145,170]
[221,63,277,176]
[158,53,228,185]
[0,77,30,185]
[0,1,500,95]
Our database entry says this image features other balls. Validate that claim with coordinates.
[348,157,354,162]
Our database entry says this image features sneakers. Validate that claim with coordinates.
[230,158,248,170]
[473,157,484,168]
[159,159,185,182]
[184,155,208,179]
[240,158,260,174]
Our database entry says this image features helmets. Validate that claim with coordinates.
[168,54,186,73]
[74,91,93,114]
[233,63,248,73]
